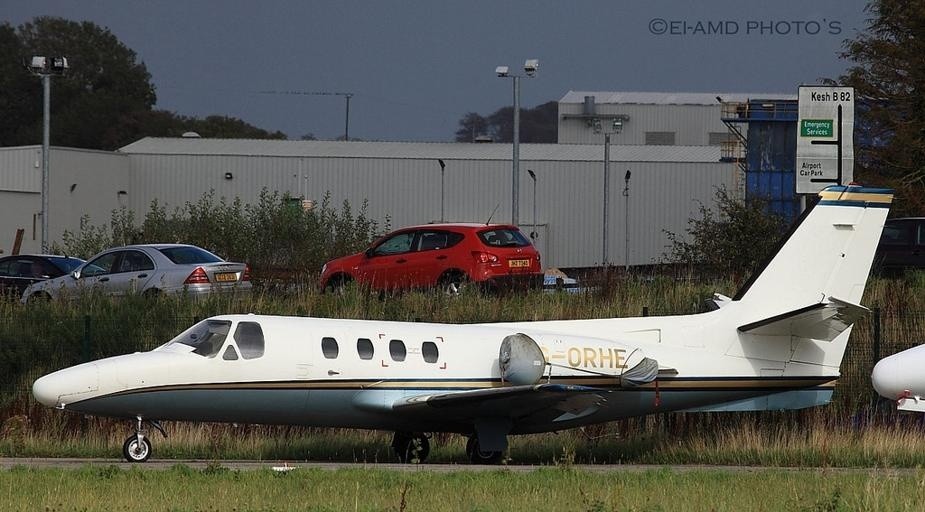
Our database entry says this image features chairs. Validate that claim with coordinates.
[420,235,441,249]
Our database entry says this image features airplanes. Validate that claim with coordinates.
[29,185,893,464]
[871,345,924,416]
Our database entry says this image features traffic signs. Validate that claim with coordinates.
[793,86,855,196]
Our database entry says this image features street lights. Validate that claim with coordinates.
[494,60,538,225]
[436,158,444,223]
[622,170,630,276]
[590,118,622,280]
[525,169,536,248]
[27,53,68,255]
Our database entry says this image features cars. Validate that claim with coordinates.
[1,254,105,304]
[878,217,924,279]
[19,242,254,313]
[318,220,540,302]
